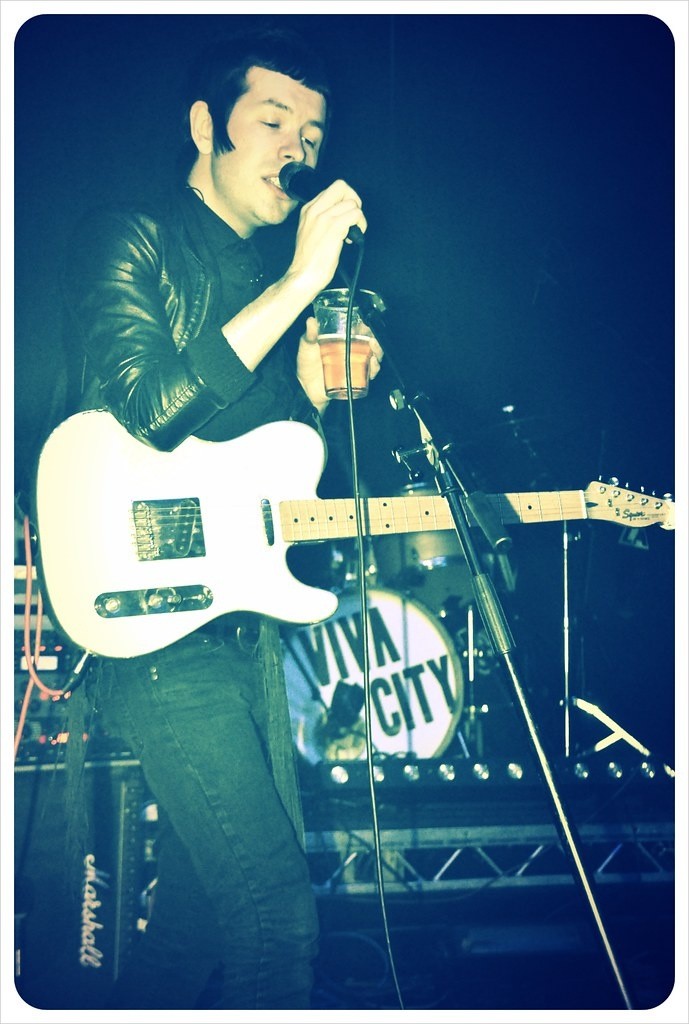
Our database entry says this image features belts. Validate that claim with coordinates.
[196,623,260,645]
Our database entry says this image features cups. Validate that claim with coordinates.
[312,281,382,401]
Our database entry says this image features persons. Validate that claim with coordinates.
[58,32,384,1009]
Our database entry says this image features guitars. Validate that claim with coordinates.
[36,410,675,661]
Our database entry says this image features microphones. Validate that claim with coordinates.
[278,161,365,249]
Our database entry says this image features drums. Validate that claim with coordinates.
[372,481,495,619]
[283,585,465,774]
